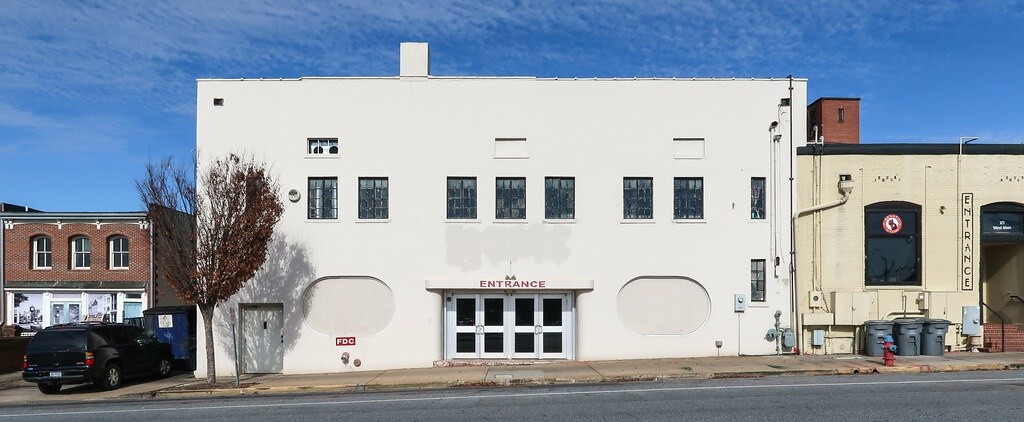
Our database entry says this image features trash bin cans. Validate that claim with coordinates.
[895,319,925,356]
[864,320,894,357]
[143,305,197,371]
[921,319,951,356]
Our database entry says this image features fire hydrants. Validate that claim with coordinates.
[880,335,898,367]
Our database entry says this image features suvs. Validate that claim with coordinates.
[20,321,178,395]
